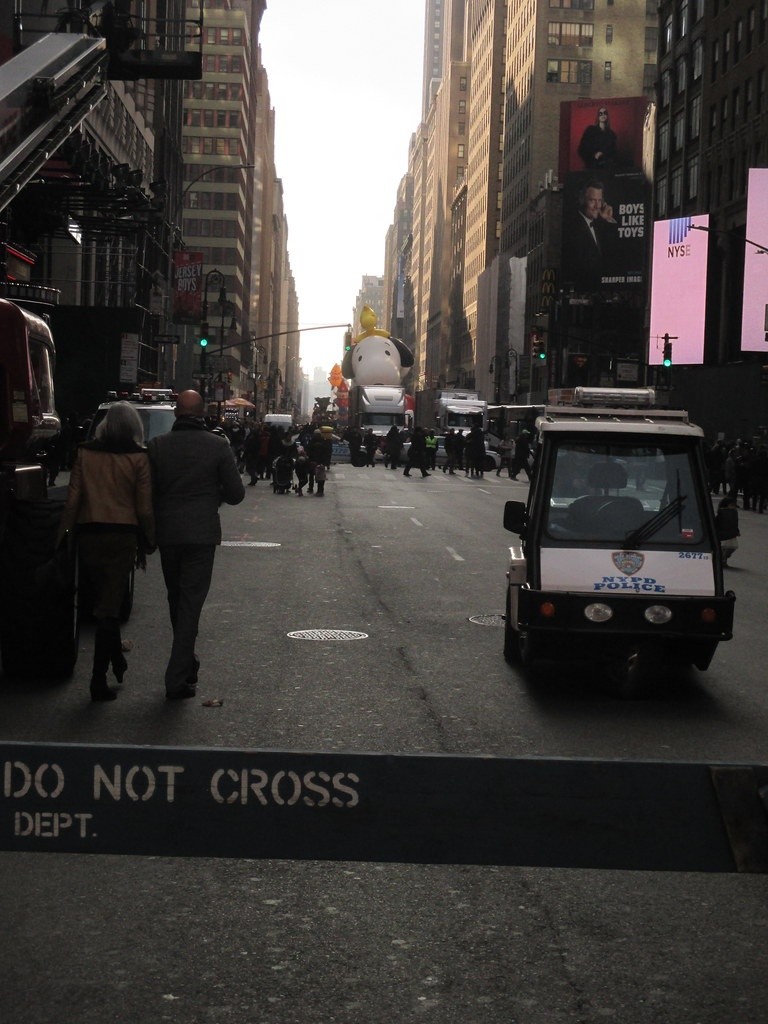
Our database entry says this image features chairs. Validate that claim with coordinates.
[566,461,644,541]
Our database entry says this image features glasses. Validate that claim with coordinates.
[598,111,609,116]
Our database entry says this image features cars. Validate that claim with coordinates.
[272,429,383,467]
[527,445,629,487]
[400,435,501,471]
[502,406,739,690]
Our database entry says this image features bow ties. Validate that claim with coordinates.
[589,221,597,227]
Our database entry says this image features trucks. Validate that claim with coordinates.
[264,414,292,432]
[414,387,488,436]
[348,385,412,444]
[548,386,665,480]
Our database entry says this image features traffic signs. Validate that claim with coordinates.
[154,334,180,346]
[194,371,214,381]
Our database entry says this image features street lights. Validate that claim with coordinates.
[489,347,518,404]
[254,345,268,421]
[200,269,228,407]
[267,360,285,414]
[217,297,237,422]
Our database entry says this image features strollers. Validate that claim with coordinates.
[270,449,295,494]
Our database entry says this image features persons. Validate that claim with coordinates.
[146,389,245,698]
[577,107,617,170]
[336,424,379,468]
[63,402,157,702]
[554,177,622,279]
[384,424,408,470]
[707,431,768,516]
[404,425,438,477]
[201,417,333,498]
[443,425,485,479]
[497,429,536,482]
[564,341,617,389]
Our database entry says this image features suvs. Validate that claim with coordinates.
[83,384,220,462]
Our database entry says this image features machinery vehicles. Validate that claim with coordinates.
[0,2,202,683]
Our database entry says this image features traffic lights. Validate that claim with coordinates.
[531,340,545,361]
[663,342,673,378]
[345,332,351,351]
[201,322,209,351]
[227,370,233,383]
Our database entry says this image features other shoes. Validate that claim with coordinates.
[314,493,325,498]
[449,472,456,475]
[294,488,303,496]
[510,477,519,481]
[165,687,196,699]
[93,688,117,702]
[443,467,446,473]
[406,474,412,476]
[186,654,201,684]
[423,474,432,477]
[114,663,129,684]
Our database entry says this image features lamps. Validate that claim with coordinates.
[13,132,168,242]
